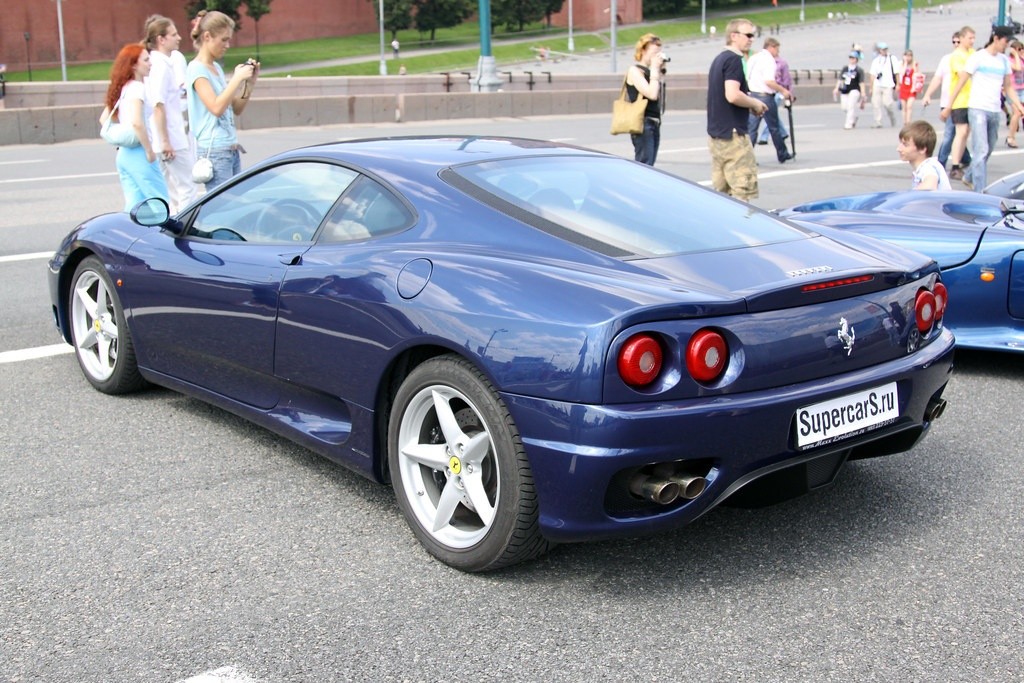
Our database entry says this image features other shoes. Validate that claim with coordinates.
[758,140,768,144]
[1005,137,1018,148]
[781,152,795,164]
[949,168,965,180]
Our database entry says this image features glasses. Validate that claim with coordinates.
[735,31,753,38]
[952,41,960,44]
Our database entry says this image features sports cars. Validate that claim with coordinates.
[757,188,1024,354]
[47,133,956,574]
[971,171,1024,228]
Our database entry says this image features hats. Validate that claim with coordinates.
[849,51,862,60]
[878,43,888,49]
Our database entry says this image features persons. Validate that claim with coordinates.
[623,33,668,167]
[739,39,795,166]
[707,18,769,208]
[100,14,197,221]
[896,120,954,191]
[832,41,920,130]
[920,25,1024,194]
[186,10,260,215]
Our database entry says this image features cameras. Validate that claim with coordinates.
[660,53,671,64]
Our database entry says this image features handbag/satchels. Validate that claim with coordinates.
[99,120,141,148]
[612,98,649,135]
[911,74,926,95]
[193,159,213,184]
